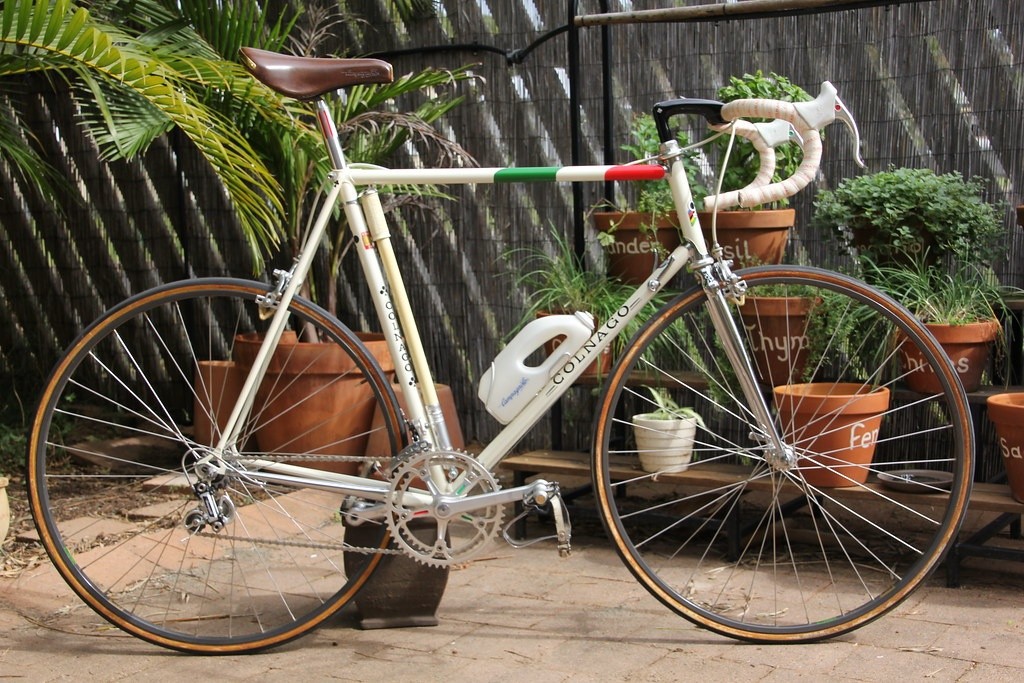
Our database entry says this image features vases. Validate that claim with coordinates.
[772,383,891,488]
[361,381,467,490]
[194,360,250,450]
[987,392,1024,504]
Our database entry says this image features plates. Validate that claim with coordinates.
[877,470,954,493]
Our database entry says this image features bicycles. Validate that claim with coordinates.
[26,36,975,658]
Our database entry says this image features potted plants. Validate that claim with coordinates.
[695,68,825,287]
[591,111,709,289]
[631,384,716,475]
[820,244,1024,395]
[489,219,684,380]
[692,251,859,389]
[73,1,407,481]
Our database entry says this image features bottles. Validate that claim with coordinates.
[477,310,595,425]
